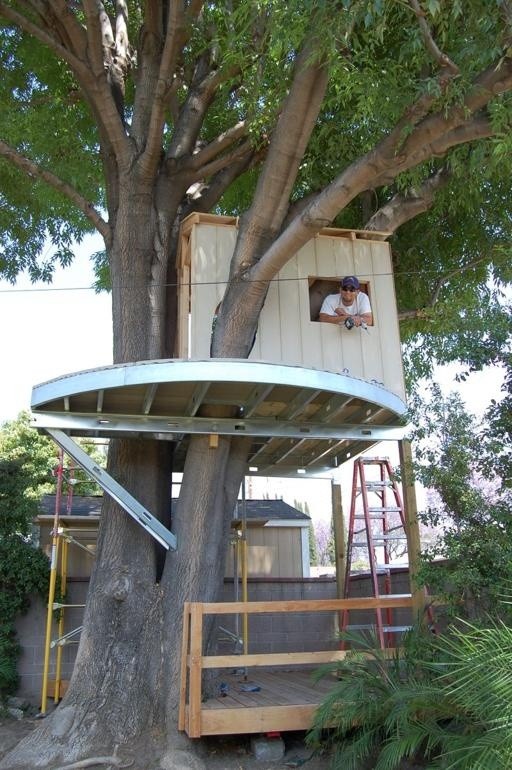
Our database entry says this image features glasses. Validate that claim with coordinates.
[343,287,355,292]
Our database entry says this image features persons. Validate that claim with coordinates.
[317,275,373,328]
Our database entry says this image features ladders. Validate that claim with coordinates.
[335,456,442,690]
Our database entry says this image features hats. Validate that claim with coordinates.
[342,276,359,289]
